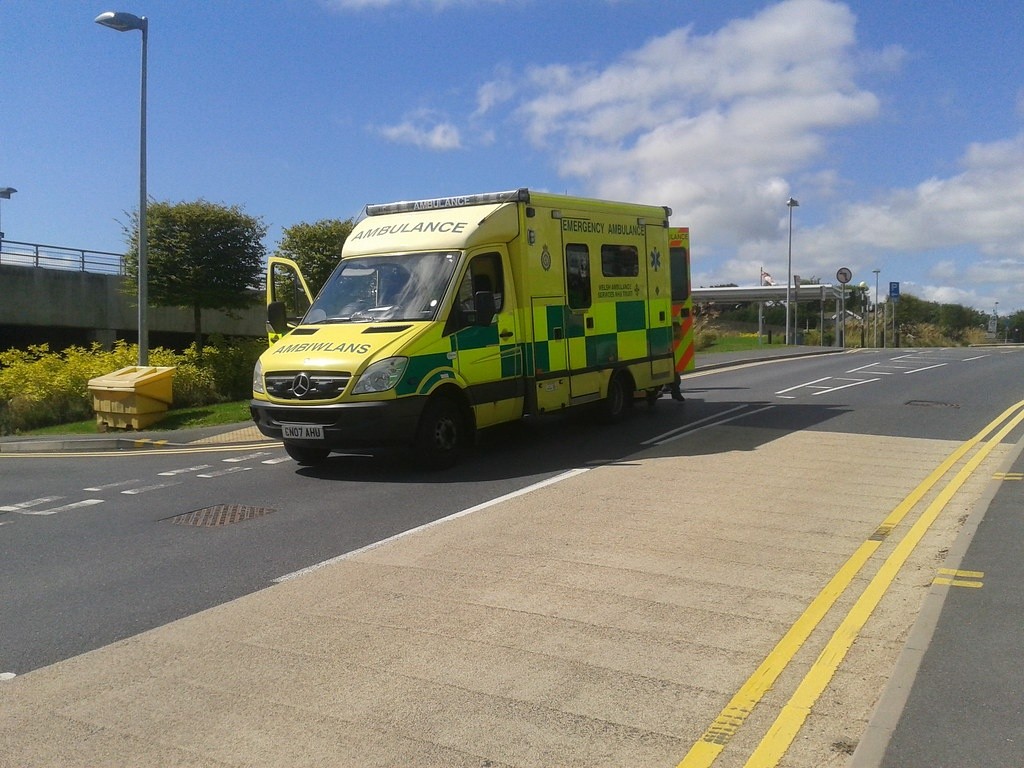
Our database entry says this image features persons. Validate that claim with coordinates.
[656,373,686,401]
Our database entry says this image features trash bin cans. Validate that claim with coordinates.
[86,364,177,432]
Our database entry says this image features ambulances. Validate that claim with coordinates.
[249,188,697,474]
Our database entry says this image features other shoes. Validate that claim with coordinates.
[672,393,685,401]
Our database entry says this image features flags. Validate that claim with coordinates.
[761,271,772,286]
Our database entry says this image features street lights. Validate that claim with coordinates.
[872,269,881,348]
[93,10,149,367]
[785,196,799,347]
